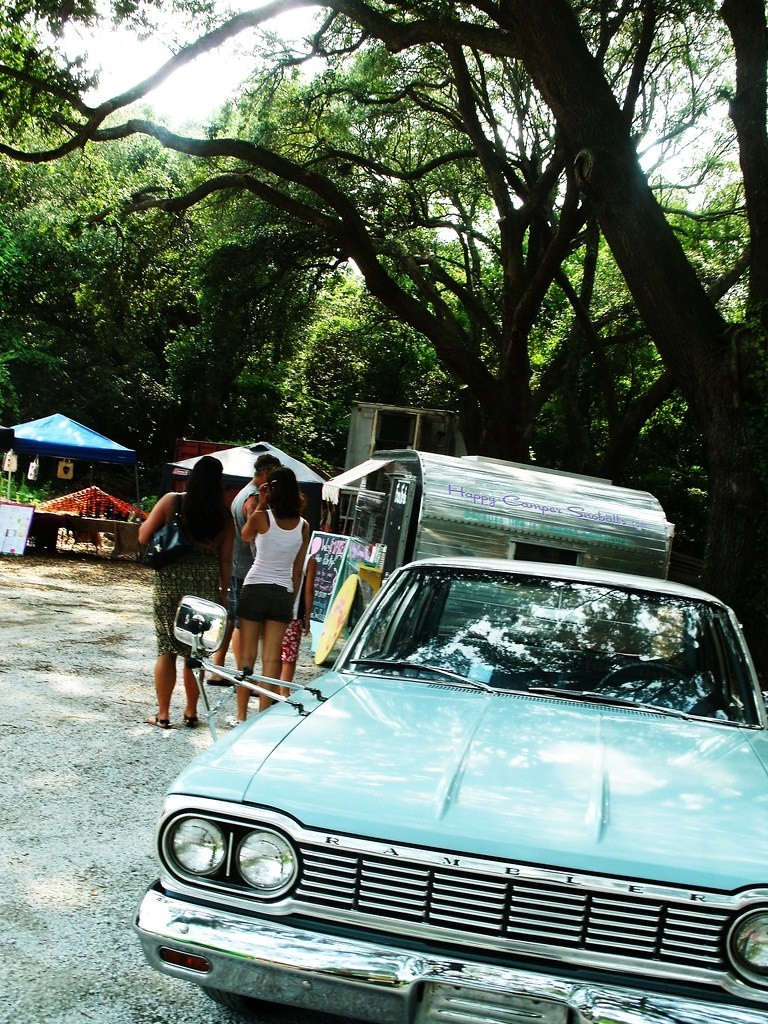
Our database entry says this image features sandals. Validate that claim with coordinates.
[183,712,198,726]
[147,713,171,730]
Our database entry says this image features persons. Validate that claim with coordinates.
[207,455,316,721]
[138,456,236,727]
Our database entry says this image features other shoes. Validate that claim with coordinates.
[226,715,243,728]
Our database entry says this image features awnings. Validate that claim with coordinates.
[322,457,395,506]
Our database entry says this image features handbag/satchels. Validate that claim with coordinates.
[27,454,39,481]
[2,449,18,472]
[142,495,194,566]
[57,457,73,480]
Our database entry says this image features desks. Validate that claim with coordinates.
[29,512,148,563]
[356,560,384,600]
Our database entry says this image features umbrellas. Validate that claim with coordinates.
[166,440,327,488]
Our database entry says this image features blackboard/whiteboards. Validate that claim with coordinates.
[0,501,35,556]
[296,531,350,623]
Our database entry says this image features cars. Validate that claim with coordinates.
[135,559,768,1023]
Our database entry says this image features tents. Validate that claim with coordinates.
[0,413,141,519]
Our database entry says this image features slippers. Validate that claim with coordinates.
[234,687,260,697]
[207,677,233,687]
